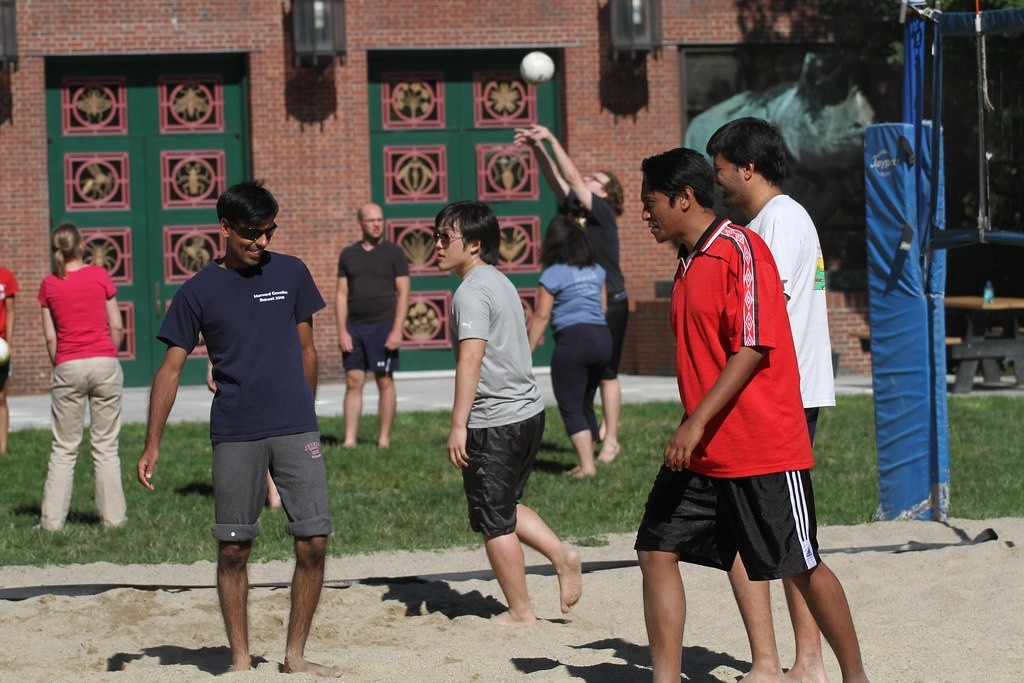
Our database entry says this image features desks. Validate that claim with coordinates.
[944,296,1024,393]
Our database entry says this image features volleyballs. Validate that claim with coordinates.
[520,51,556,84]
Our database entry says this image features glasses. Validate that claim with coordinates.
[434,233,464,246]
[229,222,277,240]
[591,176,605,187]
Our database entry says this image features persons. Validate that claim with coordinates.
[707,116,840,683]
[635,149,872,683]
[529,215,614,476]
[30,224,129,533]
[138,180,341,677]
[334,202,410,447]
[433,201,581,627]
[0,265,20,459]
[515,125,629,463]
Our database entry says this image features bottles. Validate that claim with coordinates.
[983,282,993,305]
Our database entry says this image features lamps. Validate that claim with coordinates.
[0,0,21,73]
[290,0,347,73]
[606,0,665,61]
